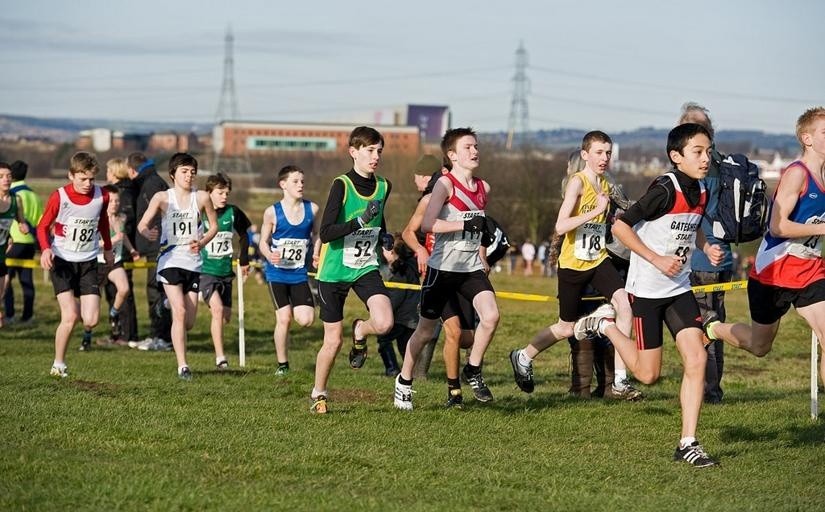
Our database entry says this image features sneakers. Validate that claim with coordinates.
[109,310,121,335]
[701,310,719,350]
[349,318,367,369]
[675,442,719,468]
[274,365,290,377]
[461,369,493,402]
[309,395,327,417]
[177,366,192,380]
[394,372,413,412]
[4,315,35,326]
[555,382,645,402]
[447,389,465,411]
[510,349,534,393]
[214,361,228,368]
[156,296,167,318]
[572,303,616,341]
[50,365,67,378]
[79,338,173,352]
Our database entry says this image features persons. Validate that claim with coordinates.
[573,123,725,468]
[258,165,322,374]
[509,102,824,400]
[310,127,499,413]
[0,161,43,329]
[106,150,252,377]
[37,152,113,377]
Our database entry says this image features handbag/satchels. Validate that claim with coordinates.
[705,152,770,242]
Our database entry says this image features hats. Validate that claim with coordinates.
[413,154,443,176]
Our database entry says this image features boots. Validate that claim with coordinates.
[379,348,401,378]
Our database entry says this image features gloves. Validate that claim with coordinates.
[382,232,395,251]
[362,200,383,224]
[464,216,486,233]
